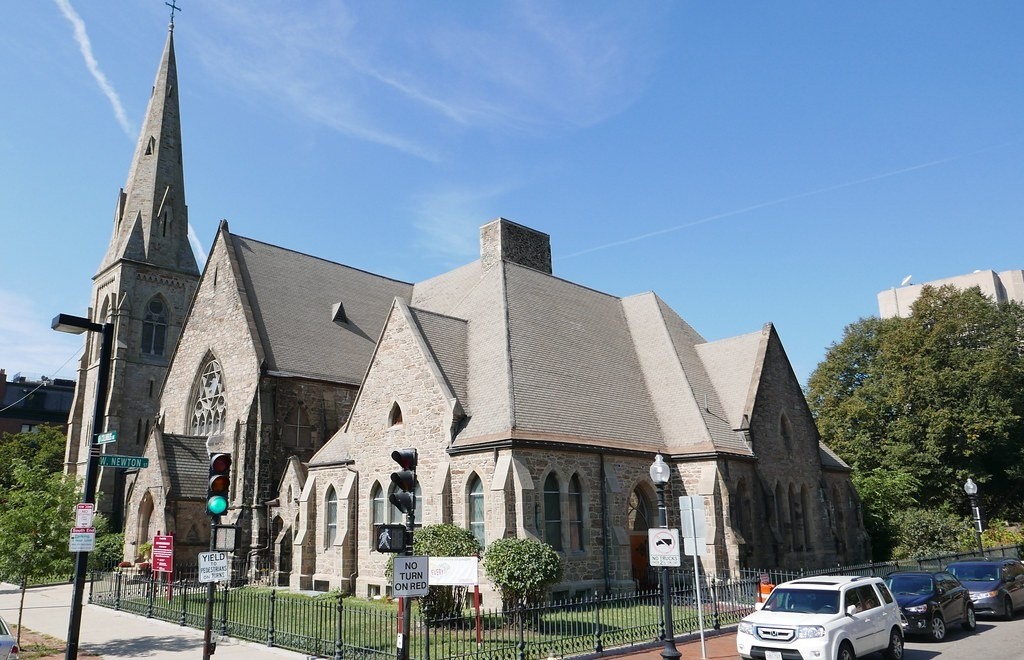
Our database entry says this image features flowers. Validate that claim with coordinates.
[118,560,131,567]
[140,563,150,569]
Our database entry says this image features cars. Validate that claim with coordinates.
[736,575,906,660]
[881,570,977,642]
[942,556,1024,619]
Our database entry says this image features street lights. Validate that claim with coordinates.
[963,477,985,558]
[49,312,119,660]
[649,453,687,660]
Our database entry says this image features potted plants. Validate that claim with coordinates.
[139,540,154,564]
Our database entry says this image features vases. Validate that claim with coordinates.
[142,567,148,578]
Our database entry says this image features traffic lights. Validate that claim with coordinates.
[205,452,233,517]
[373,523,408,553]
[214,525,243,552]
[389,447,418,511]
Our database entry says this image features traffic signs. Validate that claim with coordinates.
[97,453,150,470]
[96,430,117,445]
